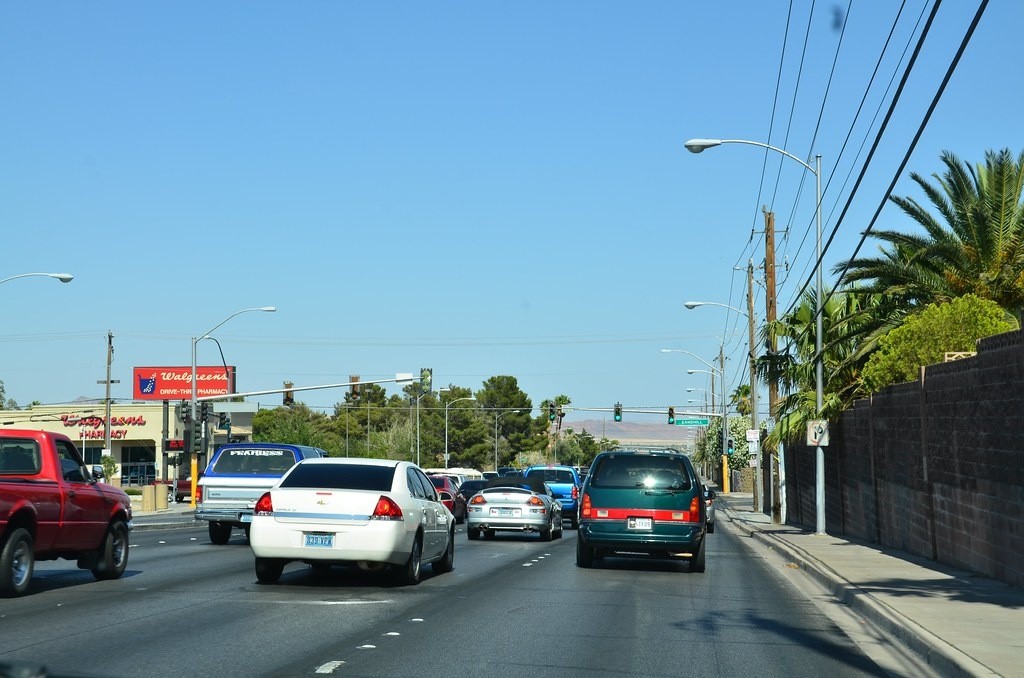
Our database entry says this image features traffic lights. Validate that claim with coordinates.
[195,420,201,452]
[727,437,735,455]
[201,402,208,422]
[668,406,674,424]
[220,412,227,425]
[180,401,190,419]
[549,404,555,421]
[614,404,623,421]
[165,439,185,452]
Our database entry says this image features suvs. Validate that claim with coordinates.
[575,446,717,573]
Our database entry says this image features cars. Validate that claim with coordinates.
[702,482,715,534]
[249,458,589,585]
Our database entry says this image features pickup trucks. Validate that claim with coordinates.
[0,429,134,599]
[193,442,330,545]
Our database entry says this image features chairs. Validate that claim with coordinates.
[2,446,35,470]
[605,467,681,486]
[557,471,569,482]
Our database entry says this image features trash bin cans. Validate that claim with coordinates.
[155,484,168,509]
[141,485,156,511]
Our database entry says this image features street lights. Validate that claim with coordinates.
[685,302,761,513]
[495,410,521,471]
[662,349,728,493]
[684,138,825,534]
[190,307,276,507]
[417,388,451,468]
